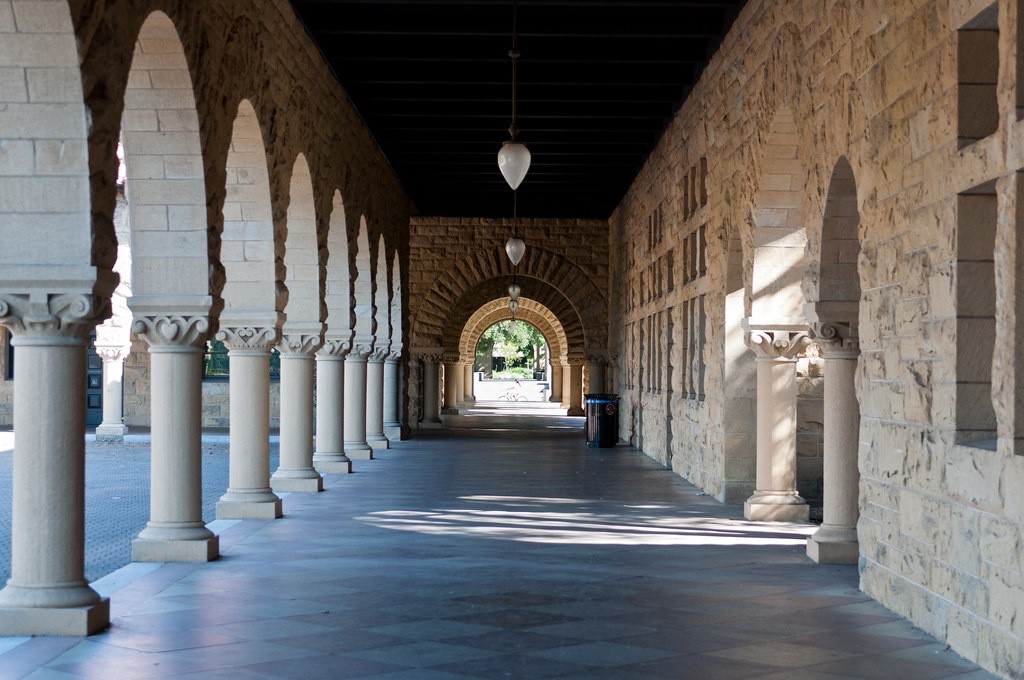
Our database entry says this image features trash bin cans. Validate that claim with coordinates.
[583,393,621,448]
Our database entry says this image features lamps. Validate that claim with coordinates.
[497,14,531,190]
[505,193,525,330]
